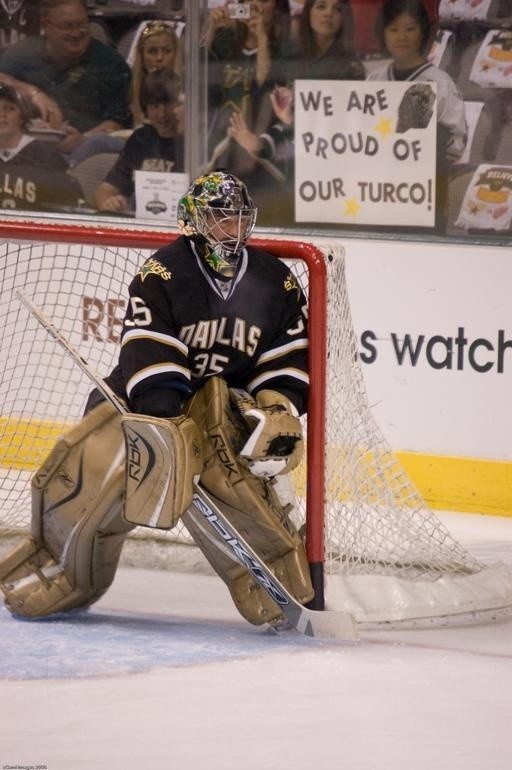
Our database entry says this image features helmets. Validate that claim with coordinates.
[178,170,254,225]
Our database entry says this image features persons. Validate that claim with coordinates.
[0,169,314,630]
[1,0,510,243]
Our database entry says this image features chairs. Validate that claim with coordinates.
[67,13,512,239]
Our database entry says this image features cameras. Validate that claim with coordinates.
[228,3,251,19]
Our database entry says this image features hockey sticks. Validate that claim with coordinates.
[14,288,356,643]
